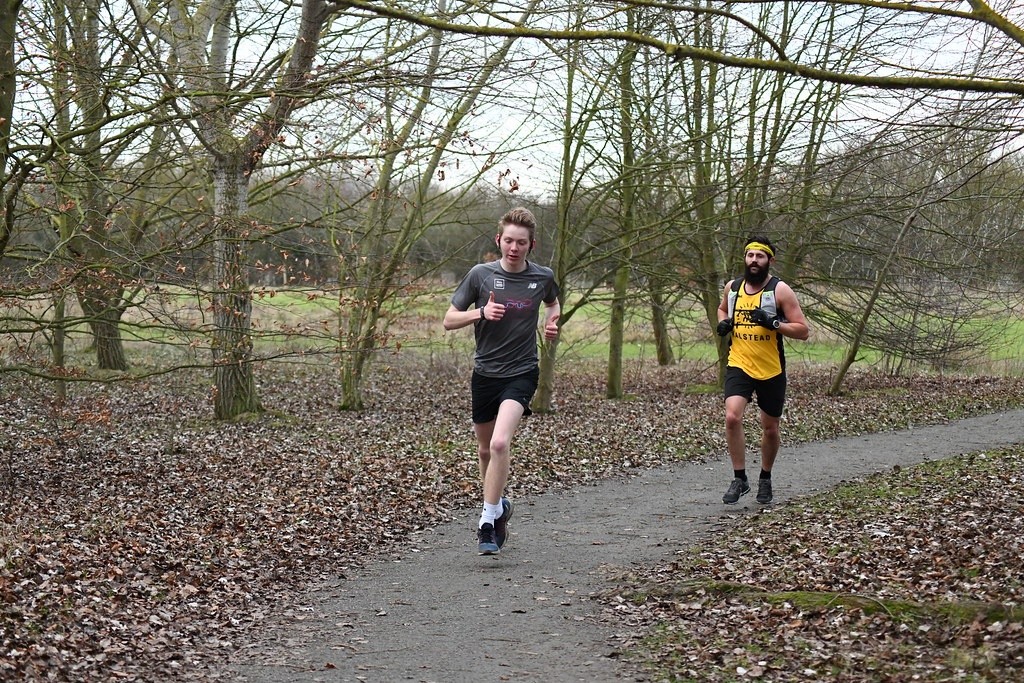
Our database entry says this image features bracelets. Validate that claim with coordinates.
[480,305,486,320]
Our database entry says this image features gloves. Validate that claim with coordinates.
[755,306,775,332]
[717,319,732,337]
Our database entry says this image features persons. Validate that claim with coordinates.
[442,207,561,557]
[716,236,809,503]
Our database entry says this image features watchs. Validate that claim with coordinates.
[770,319,780,331]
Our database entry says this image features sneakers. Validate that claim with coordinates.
[477,499,514,556]
[756,474,773,505]
[722,476,749,506]
